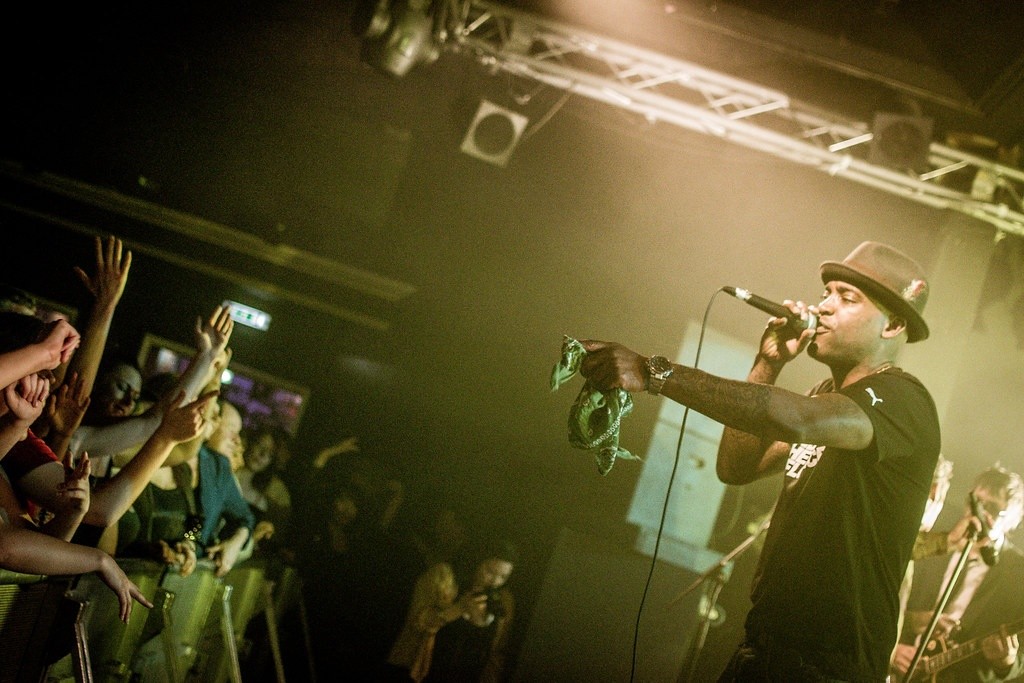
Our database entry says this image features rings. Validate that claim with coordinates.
[198,408,204,415]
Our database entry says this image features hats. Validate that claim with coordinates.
[819,241,928,343]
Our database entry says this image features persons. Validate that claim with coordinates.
[0,235,516,683]
[576,240,942,683]
[887,453,1024,683]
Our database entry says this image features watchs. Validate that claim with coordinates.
[644,355,674,396]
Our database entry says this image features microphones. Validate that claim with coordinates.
[969,491,1001,566]
[723,286,819,333]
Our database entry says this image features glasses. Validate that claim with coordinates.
[967,495,1019,519]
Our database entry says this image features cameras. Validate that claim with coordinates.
[471,585,507,620]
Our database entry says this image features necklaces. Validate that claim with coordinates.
[874,366,893,374]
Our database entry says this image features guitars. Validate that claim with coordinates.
[891,616,1024,683]
